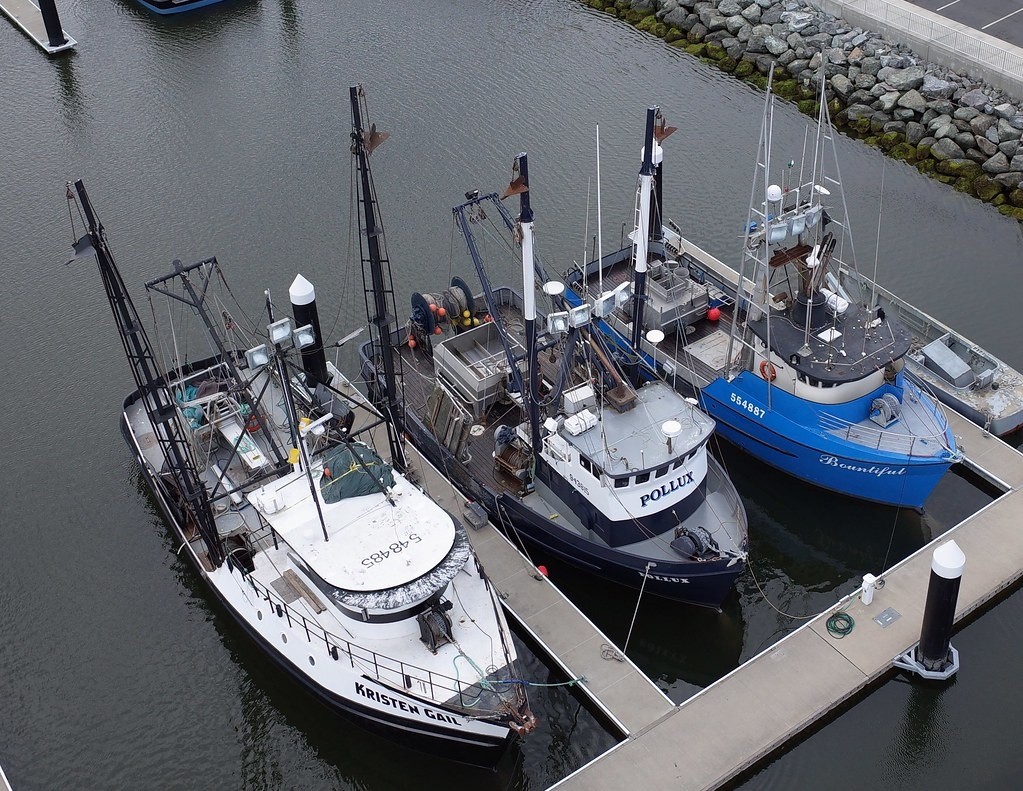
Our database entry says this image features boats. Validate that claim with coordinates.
[563,40,966,510]
[345,83,751,611]
[60,176,536,774]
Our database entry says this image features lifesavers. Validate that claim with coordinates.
[759,361,776,382]
